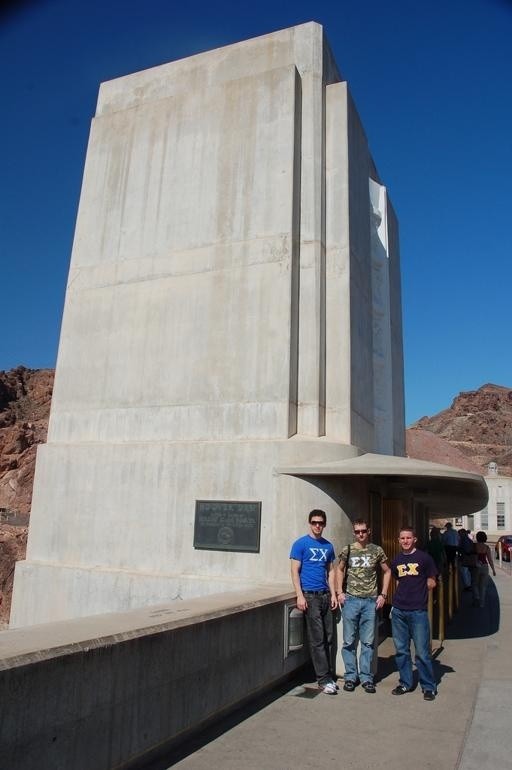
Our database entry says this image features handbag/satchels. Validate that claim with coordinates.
[462,557,482,567]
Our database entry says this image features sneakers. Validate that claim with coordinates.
[362,683,376,693]
[425,691,435,700]
[319,680,339,693]
[392,685,412,695]
[343,681,355,691]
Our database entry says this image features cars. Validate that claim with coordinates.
[495,535,512,561]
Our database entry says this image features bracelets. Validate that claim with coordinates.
[380,594,387,599]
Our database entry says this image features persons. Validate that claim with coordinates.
[389,527,437,700]
[427,522,496,608]
[338,518,392,693]
[290,508,340,694]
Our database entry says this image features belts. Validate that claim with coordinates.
[303,591,328,595]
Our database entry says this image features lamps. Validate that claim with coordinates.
[284,602,305,660]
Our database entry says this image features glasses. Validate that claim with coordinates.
[310,522,325,527]
[356,531,369,534]
